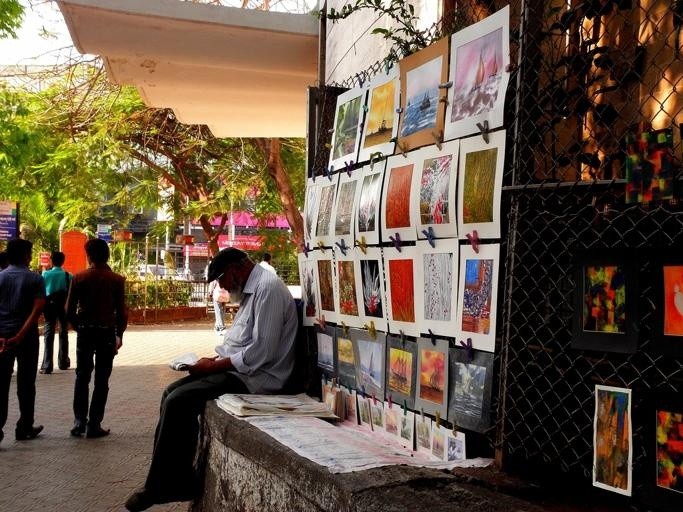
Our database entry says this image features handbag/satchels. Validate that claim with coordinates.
[41,290,67,319]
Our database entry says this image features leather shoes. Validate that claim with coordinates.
[58,362,69,370]
[71,423,109,439]
[14,423,43,441]
[38,365,53,374]
[124,483,203,512]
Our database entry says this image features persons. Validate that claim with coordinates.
[201,255,212,281]
[123,245,299,511]
[0,239,44,443]
[207,279,227,331]
[40,251,72,374]
[66,238,129,439]
[259,253,276,275]
[0,251,9,271]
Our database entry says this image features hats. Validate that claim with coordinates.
[206,247,247,283]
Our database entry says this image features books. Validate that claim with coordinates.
[215,391,331,418]
